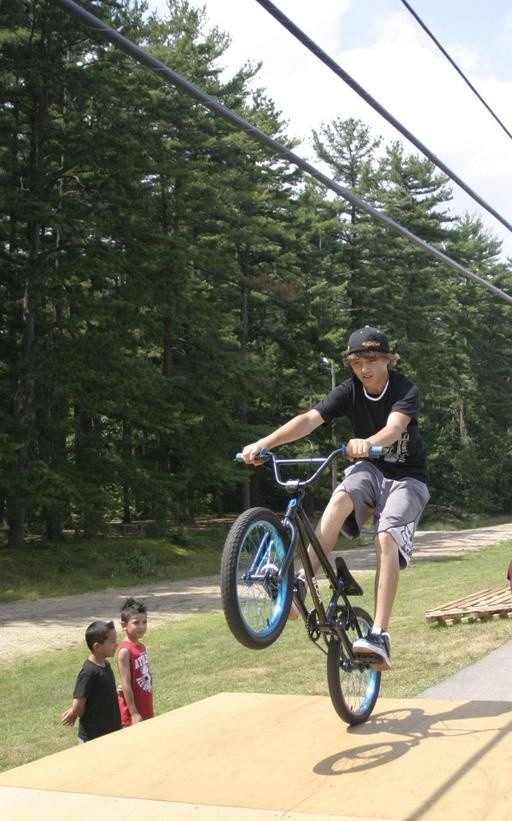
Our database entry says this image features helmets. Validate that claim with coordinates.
[346,327,390,358]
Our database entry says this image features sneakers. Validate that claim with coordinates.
[259,564,308,621]
[352,627,392,671]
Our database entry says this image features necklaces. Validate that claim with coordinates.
[361,381,389,403]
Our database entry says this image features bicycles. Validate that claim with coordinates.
[218,444,383,725]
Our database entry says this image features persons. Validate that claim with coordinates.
[59,620,122,744]
[240,323,432,671]
[507,561,511,594]
[114,598,154,725]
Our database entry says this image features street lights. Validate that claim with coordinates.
[322,357,338,492]
